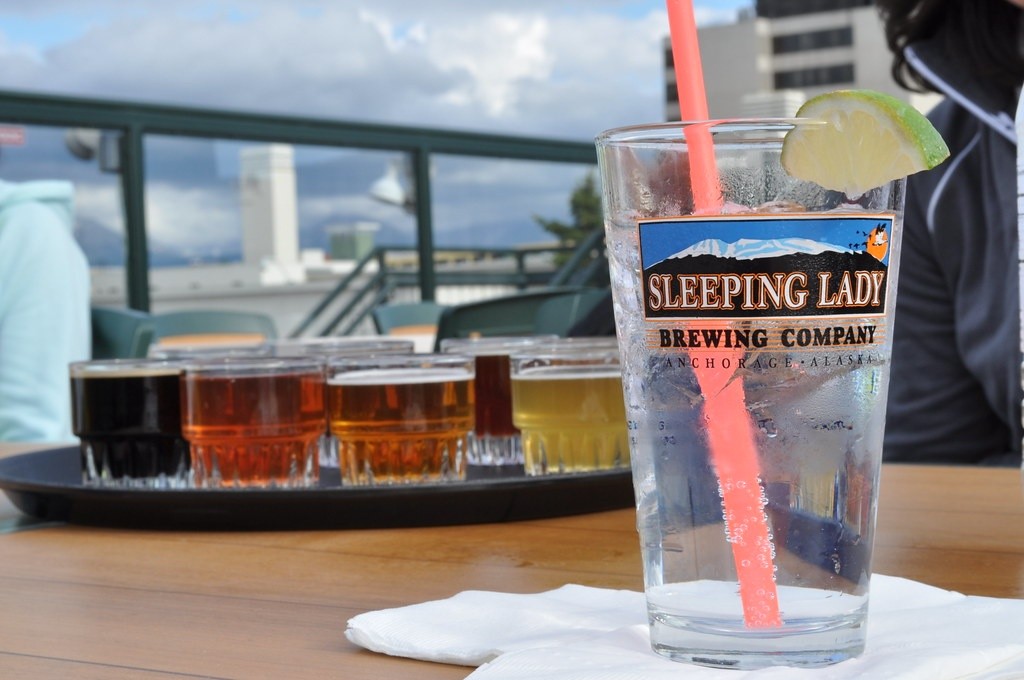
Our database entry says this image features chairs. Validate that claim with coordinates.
[87,284,609,360]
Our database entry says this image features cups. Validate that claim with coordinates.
[67,335,623,486]
[595,117,906,670]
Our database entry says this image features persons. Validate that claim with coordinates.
[820,0,1024,469]
[0,180,93,517]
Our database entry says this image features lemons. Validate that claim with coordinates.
[780,88,950,198]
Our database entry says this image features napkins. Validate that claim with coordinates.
[343,573,1023,679]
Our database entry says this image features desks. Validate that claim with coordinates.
[0,441,1024,679]
